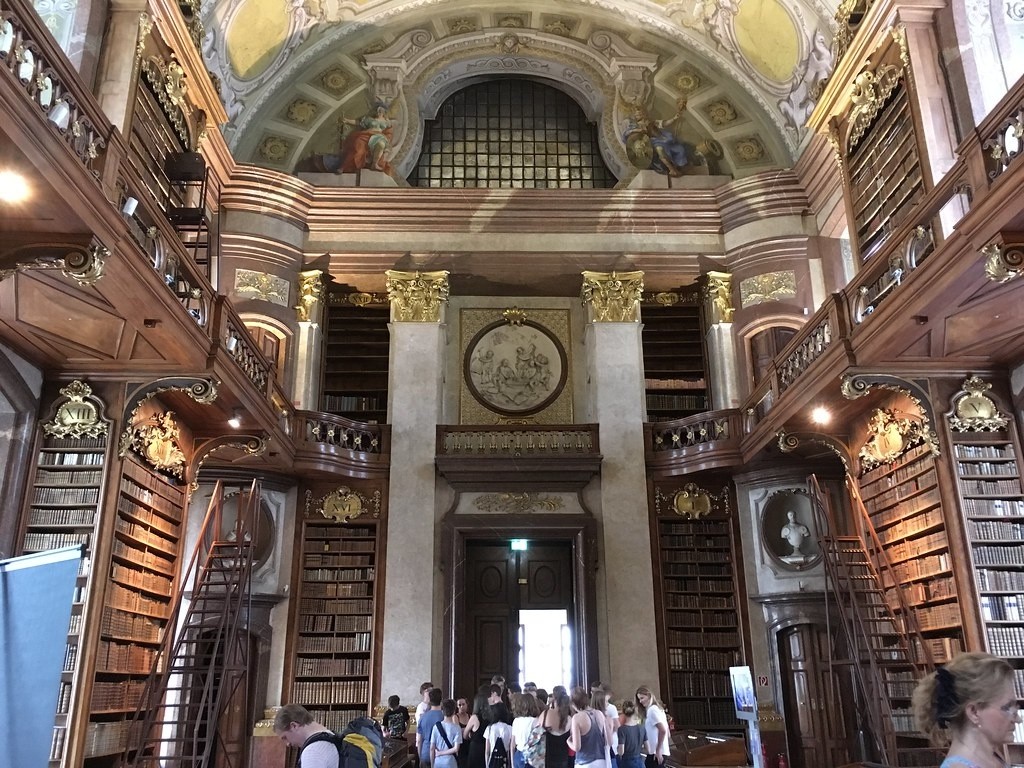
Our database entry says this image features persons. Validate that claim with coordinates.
[414,675,671,768]
[342,107,393,171]
[381,695,411,739]
[781,510,811,556]
[625,103,687,176]
[273,703,340,768]
[911,651,1023,768]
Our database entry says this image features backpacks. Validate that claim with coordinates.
[523,710,552,768]
[489,724,509,768]
[300,730,375,768]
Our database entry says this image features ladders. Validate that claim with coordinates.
[806,469,949,767]
[123,477,261,768]
[163,150,221,321]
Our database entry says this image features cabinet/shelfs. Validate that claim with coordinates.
[11,373,1024,768]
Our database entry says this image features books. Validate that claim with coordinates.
[645,379,709,422]
[659,522,746,725]
[848,82,935,309]
[123,77,184,221]
[859,443,1024,742]
[19,435,184,761]
[323,395,377,424]
[292,527,375,734]
